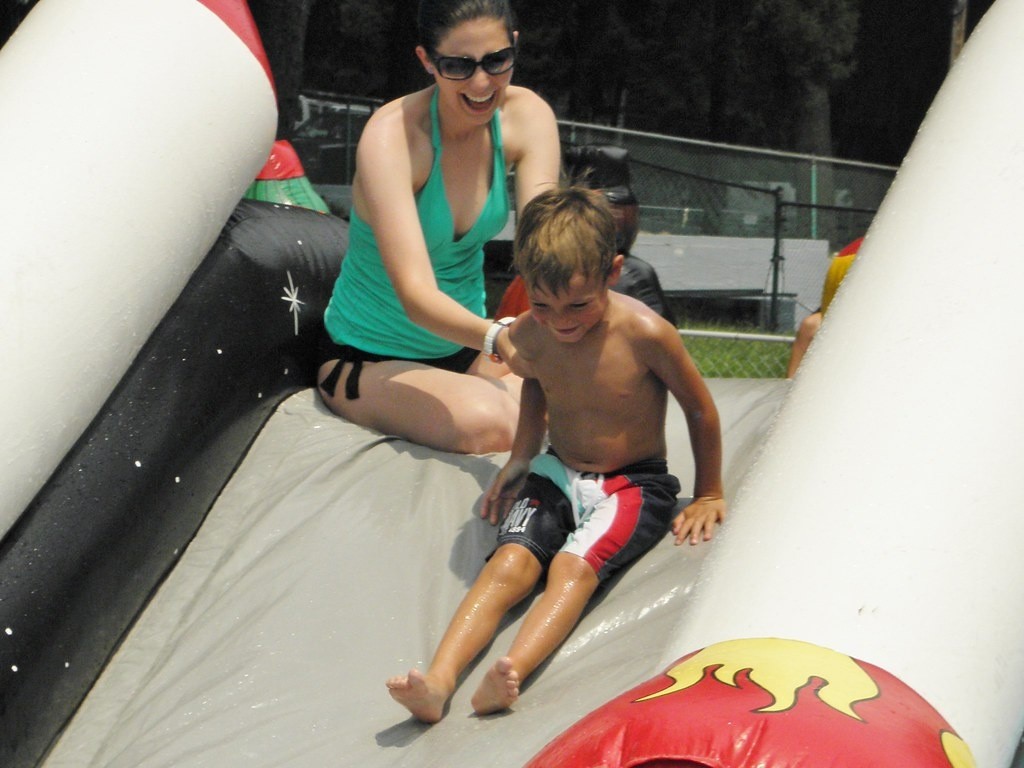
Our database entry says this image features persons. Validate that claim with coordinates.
[788,307,822,380]
[304,0,561,455]
[383,184,726,723]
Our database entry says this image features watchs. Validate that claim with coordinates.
[482,317,516,365]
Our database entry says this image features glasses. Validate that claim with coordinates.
[422,42,516,80]
